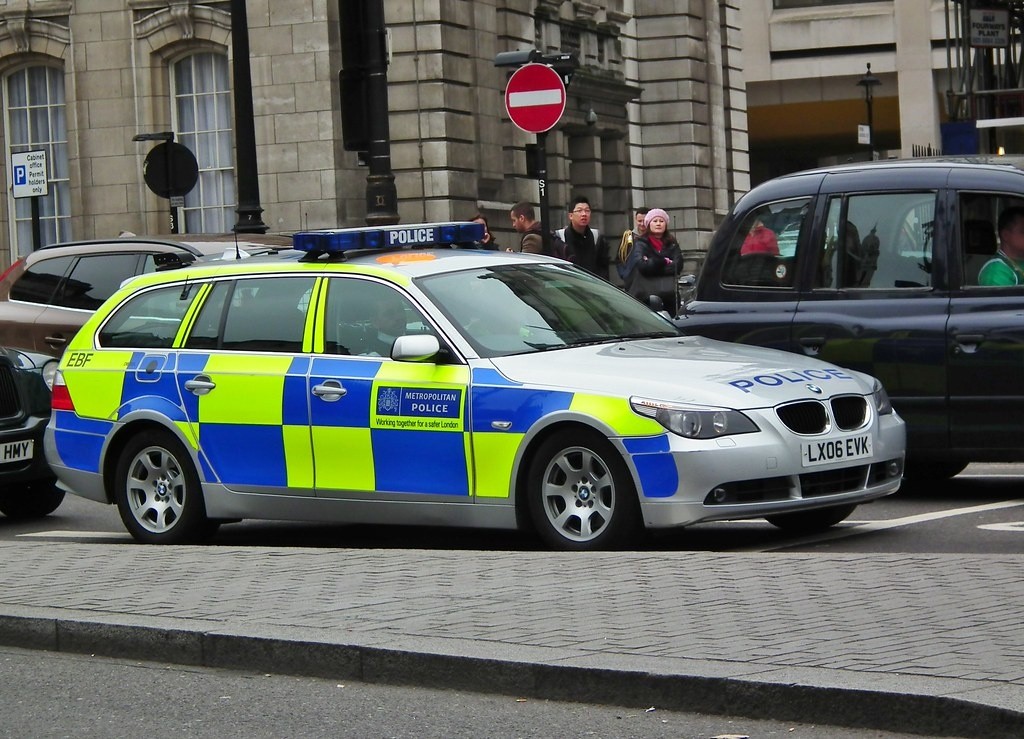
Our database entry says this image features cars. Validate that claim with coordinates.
[0,344,68,525]
[777,221,838,258]
[44,222,908,551]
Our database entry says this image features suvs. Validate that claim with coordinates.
[648,154,1024,491]
[0,232,262,357]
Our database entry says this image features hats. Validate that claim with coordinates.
[644,209,669,228]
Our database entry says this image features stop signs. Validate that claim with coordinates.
[504,62,566,135]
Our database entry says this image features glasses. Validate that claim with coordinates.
[571,208,592,215]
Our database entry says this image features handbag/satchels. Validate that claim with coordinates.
[628,270,677,304]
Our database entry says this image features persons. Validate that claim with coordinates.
[506,200,543,254]
[977,207,1024,286]
[355,292,406,357]
[616,207,650,278]
[740,220,780,255]
[468,215,500,251]
[555,196,609,281]
[624,208,684,318]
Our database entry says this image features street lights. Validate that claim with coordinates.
[857,62,882,161]
